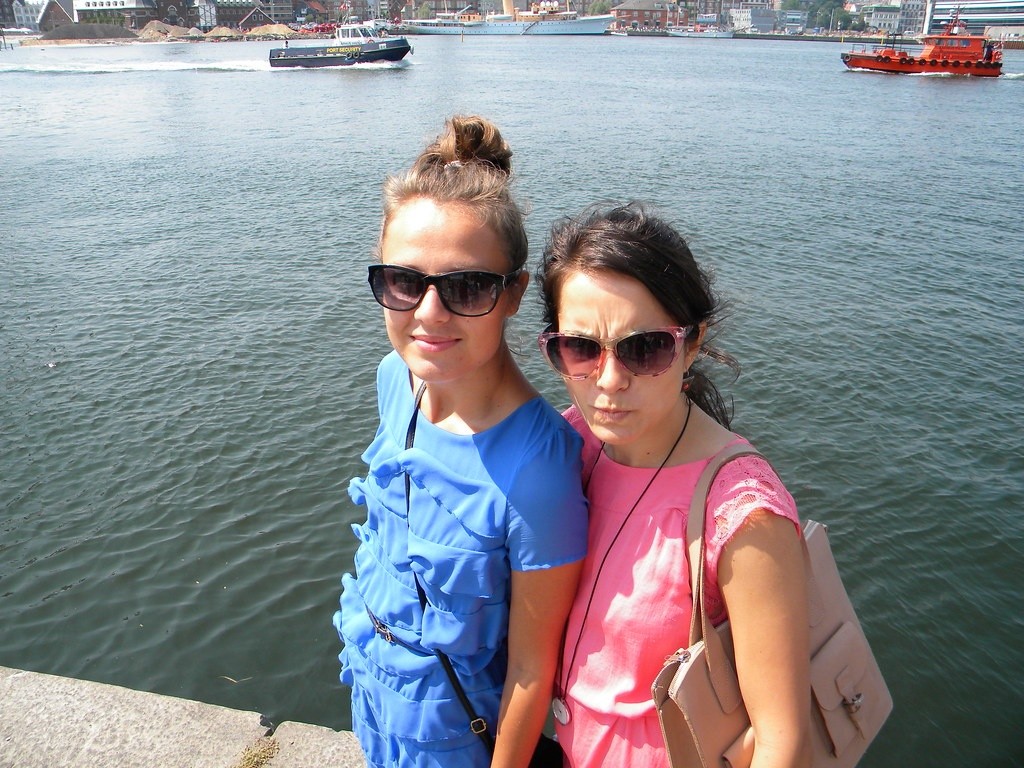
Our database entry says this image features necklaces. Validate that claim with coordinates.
[552,393,691,724]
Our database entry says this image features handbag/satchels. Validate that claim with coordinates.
[650,442,895,768]
[487,722,565,767]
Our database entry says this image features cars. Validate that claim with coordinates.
[288,21,318,33]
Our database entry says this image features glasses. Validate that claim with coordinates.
[368,264,523,318]
[537,325,697,382]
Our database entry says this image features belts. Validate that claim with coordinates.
[365,605,434,656]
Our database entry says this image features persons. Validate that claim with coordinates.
[534,198,894,768]
[983,41,1002,62]
[334,116,589,768]
[381,28,388,38]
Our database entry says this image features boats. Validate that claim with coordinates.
[840,5,1004,77]
[377,4,618,35]
[269,22,412,68]
[666,28,735,38]
